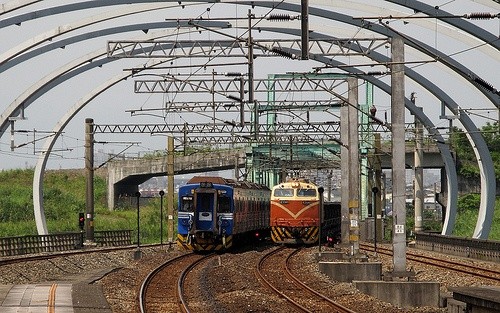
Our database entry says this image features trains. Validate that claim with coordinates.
[178,176,271,255]
[271,172,341,244]
